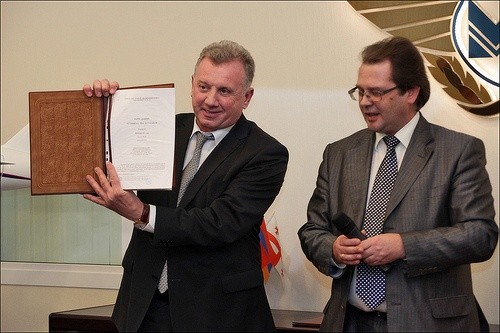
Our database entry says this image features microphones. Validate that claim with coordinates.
[330,211,393,272]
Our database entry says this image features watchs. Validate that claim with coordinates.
[134,205,150,229]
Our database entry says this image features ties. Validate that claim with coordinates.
[354,135,401,311]
[154,130,215,293]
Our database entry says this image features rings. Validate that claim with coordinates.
[341,254,344,260]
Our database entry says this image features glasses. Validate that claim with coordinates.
[347,85,400,106]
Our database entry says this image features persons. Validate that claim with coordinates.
[82,40,288,333]
[297,36,498,332]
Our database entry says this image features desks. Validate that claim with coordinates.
[49,304,500,333]
